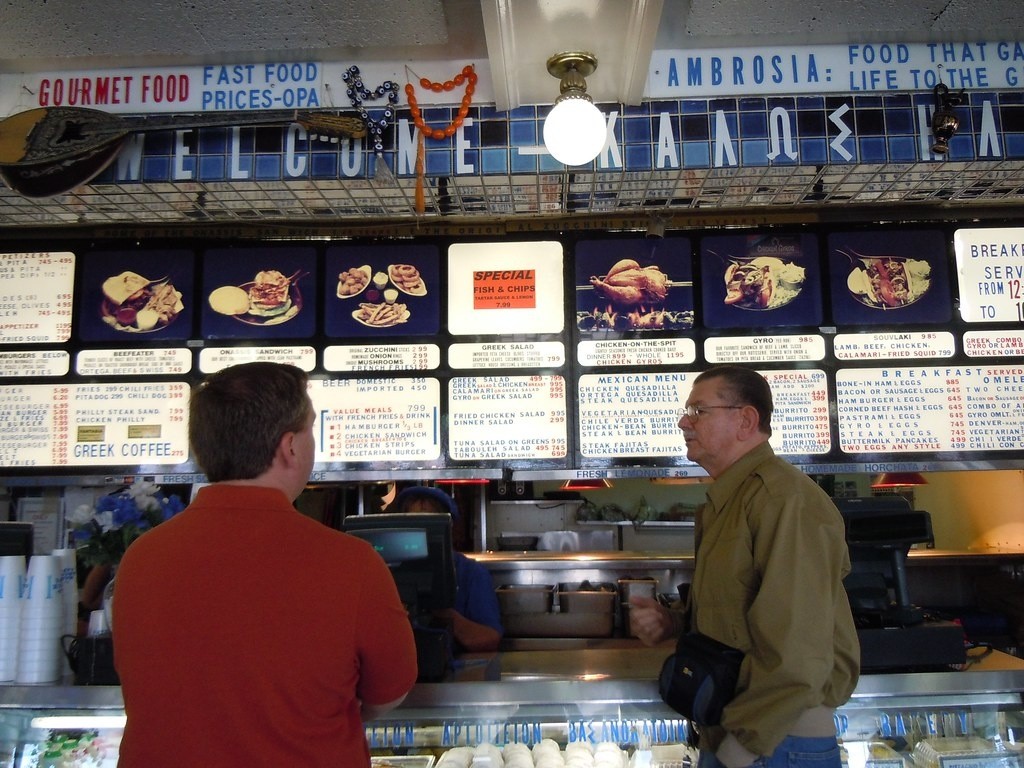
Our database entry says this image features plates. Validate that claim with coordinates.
[103,287,175,332]
[388,265,427,296]
[336,265,372,299]
[232,280,302,328]
[352,307,410,327]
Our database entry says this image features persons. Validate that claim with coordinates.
[83,361,417,768]
[384,484,505,655]
[628,364,860,768]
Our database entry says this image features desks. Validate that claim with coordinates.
[905,547,1024,568]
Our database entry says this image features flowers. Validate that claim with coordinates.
[66,482,188,565]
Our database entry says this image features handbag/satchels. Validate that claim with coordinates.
[657,631,746,727]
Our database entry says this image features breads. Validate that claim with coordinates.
[209,286,250,316]
[102,271,150,306]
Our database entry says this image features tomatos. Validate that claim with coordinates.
[406,66,477,140]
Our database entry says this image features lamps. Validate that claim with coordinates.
[871,474,929,488]
[561,479,613,490]
[542,51,607,166]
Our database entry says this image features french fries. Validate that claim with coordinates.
[357,303,409,324]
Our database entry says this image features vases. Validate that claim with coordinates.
[104,563,118,632]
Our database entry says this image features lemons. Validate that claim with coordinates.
[847,267,868,294]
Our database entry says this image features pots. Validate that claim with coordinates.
[837,242,926,309]
[704,248,802,311]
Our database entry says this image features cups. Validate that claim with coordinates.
[0,549,78,688]
[384,290,398,303]
[374,274,388,289]
[89,610,107,636]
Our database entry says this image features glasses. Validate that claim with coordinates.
[677,405,744,423]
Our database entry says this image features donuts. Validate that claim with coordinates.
[391,264,424,293]
